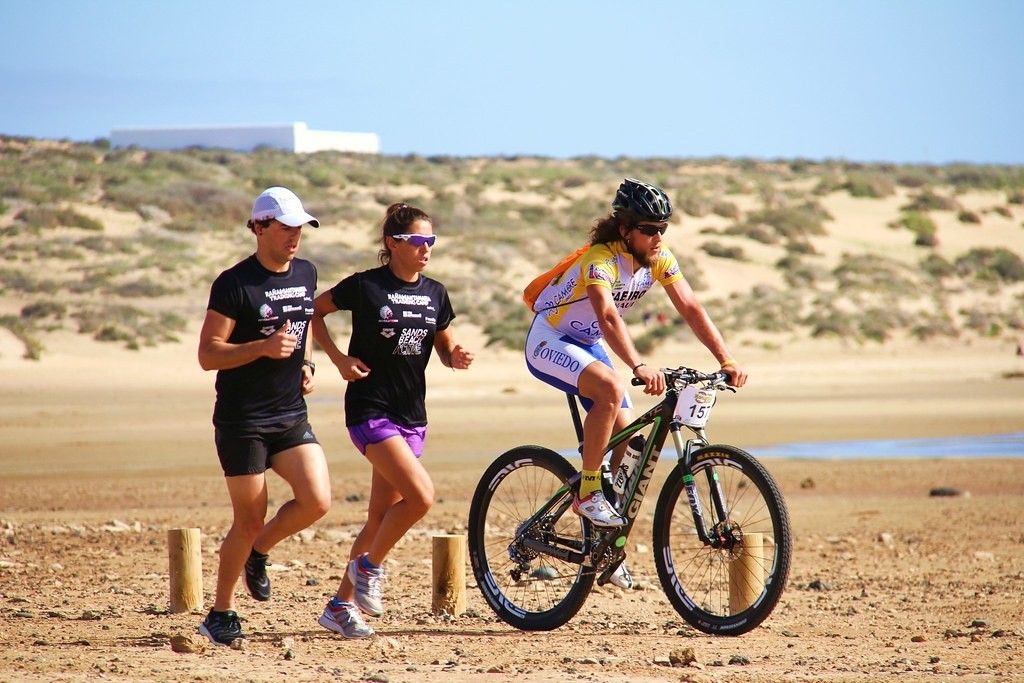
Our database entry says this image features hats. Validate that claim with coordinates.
[251,187,320,228]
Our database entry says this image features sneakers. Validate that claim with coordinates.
[242,547,272,602]
[589,556,633,589]
[572,490,628,526]
[199,607,248,646]
[346,553,384,618]
[318,596,375,639]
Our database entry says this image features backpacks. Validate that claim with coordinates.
[524,243,618,312]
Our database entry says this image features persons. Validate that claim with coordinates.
[524,179,748,588]
[197,186,331,645]
[314,202,475,640]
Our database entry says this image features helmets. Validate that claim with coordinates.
[613,178,673,220]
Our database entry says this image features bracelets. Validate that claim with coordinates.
[447,351,455,372]
[632,363,646,374]
[721,360,737,366]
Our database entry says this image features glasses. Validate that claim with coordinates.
[392,234,437,247]
[632,222,668,237]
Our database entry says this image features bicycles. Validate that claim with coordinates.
[467,366,792,637]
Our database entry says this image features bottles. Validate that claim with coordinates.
[612,433,647,495]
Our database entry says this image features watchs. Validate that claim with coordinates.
[302,358,316,375]
